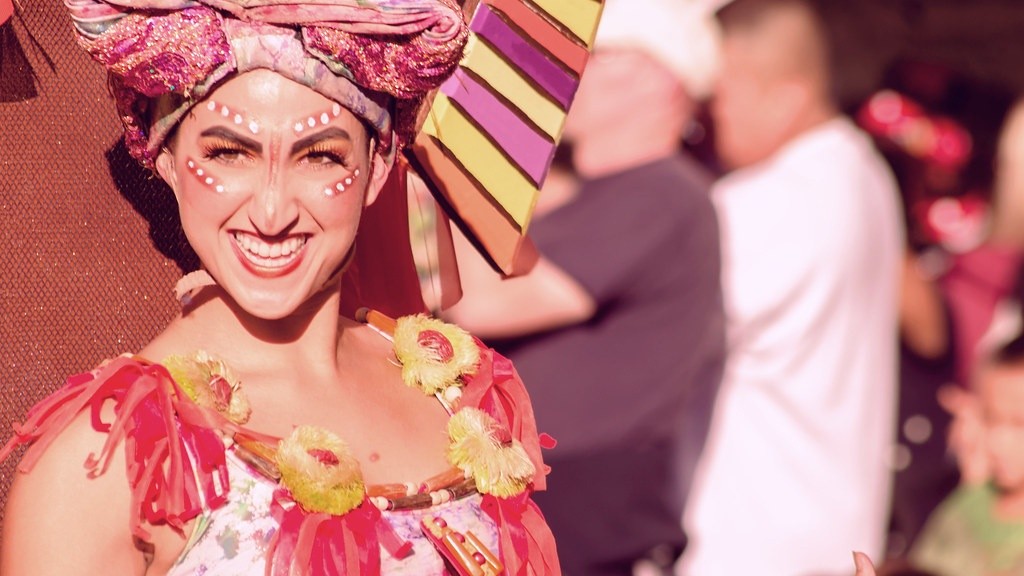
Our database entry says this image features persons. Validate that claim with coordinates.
[903,91,1024,576]
[430,42,729,575]
[0,1,562,575]
[672,0,906,576]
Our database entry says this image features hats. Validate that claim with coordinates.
[592,1,725,100]
[63,0,473,184]
